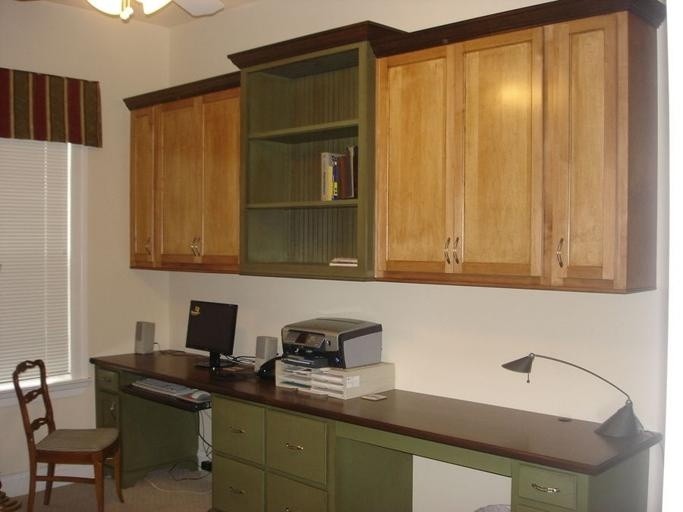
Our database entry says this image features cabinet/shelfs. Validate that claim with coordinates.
[159,72,241,272]
[122,97,159,269]
[537,0,664,293]
[373,7,537,285]
[225,20,424,283]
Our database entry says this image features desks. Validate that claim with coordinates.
[85,347,663,511]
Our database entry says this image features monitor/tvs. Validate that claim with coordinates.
[185,300,238,368]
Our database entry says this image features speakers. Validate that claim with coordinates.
[135,321,155,354]
[256,335,278,373]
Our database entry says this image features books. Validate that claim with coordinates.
[329,258,357,267]
[319,145,357,201]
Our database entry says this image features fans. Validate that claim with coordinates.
[173,1,224,18]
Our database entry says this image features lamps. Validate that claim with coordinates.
[501,350,642,439]
[84,0,176,21]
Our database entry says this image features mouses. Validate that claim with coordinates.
[192,390,212,400]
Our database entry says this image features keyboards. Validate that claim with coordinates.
[131,377,199,397]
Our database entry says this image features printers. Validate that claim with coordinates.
[281,319,382,369]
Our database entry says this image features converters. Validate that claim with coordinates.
[200,460,212,472]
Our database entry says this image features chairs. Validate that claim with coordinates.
[10,358,125,511]
